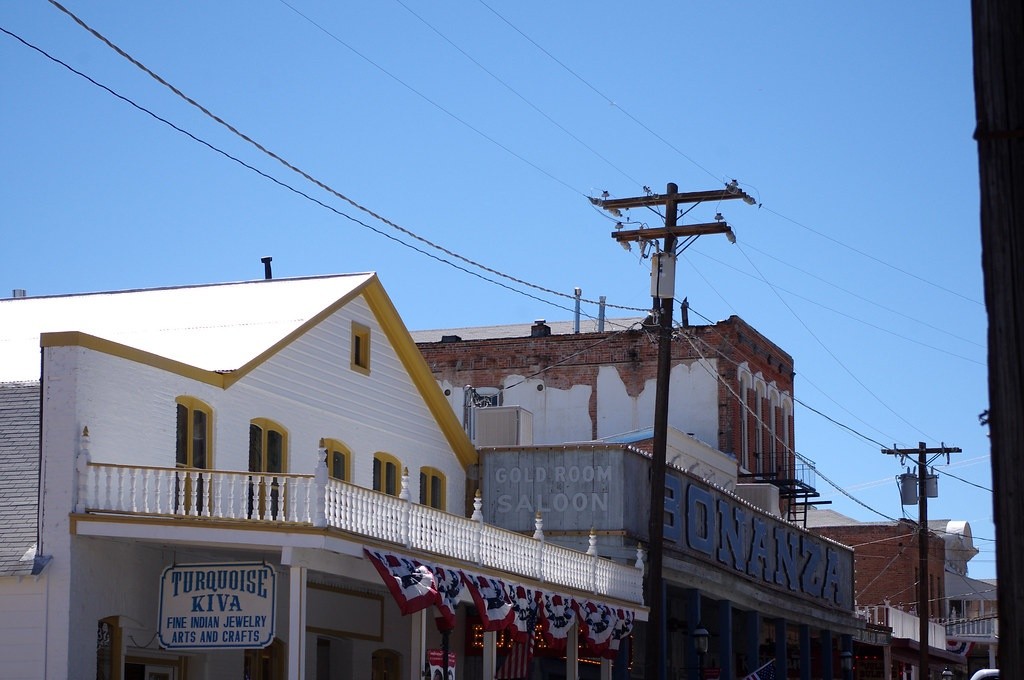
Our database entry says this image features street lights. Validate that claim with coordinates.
[692,621,709,680]
[840,648,852,680]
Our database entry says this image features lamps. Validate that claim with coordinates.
[693,622,711,655]
[942,668,954,680]
[841,649,852,673]
